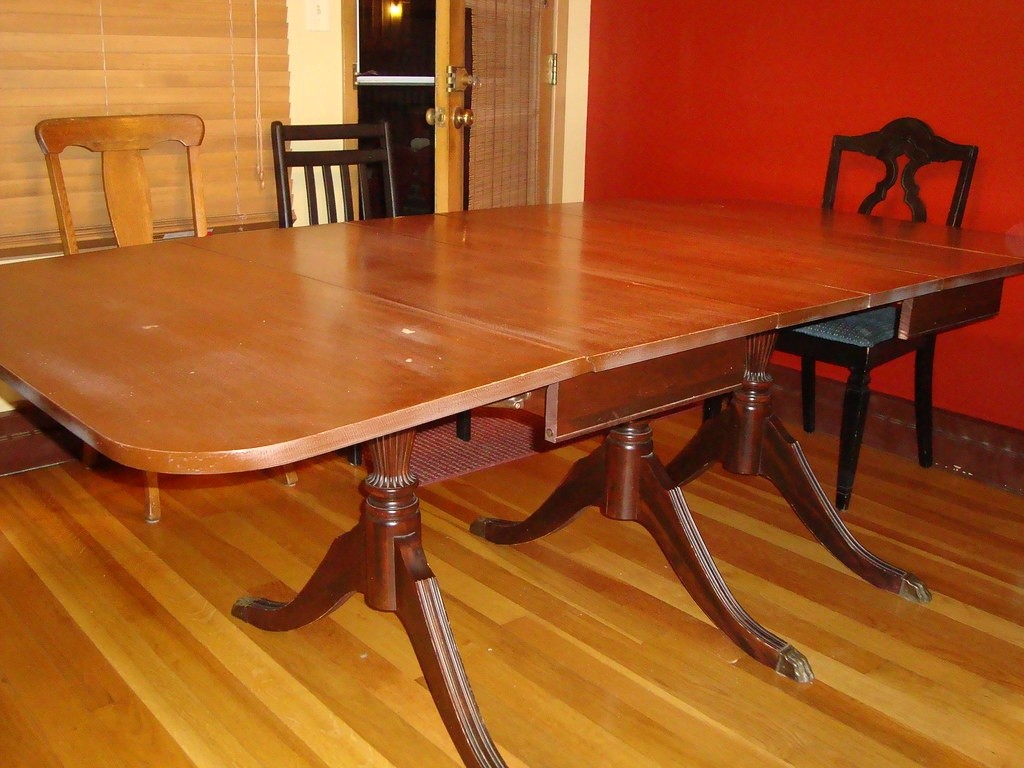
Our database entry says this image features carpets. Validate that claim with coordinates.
[359,407,547,485]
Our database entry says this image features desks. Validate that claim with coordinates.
[0,200,1024,768]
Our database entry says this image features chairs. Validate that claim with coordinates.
[703,118,978,510]
[272,118,472,465]
[36,112,211,523]
